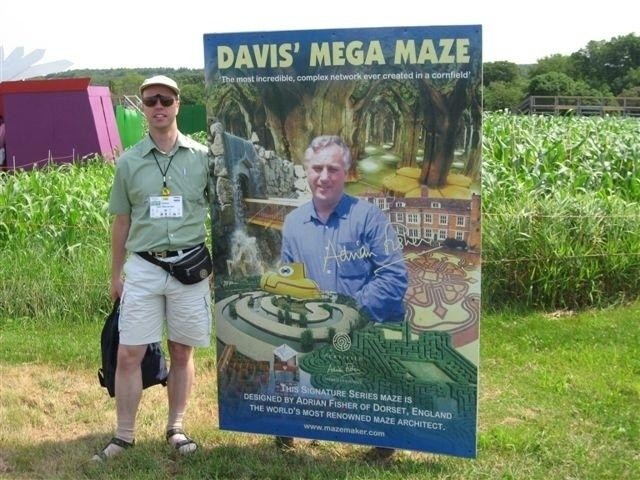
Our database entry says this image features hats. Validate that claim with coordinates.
[139,75,180,95]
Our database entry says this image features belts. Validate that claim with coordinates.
[149,249,191,259]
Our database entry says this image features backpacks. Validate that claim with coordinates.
[96,295,170,397]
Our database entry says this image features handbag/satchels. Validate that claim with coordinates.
[165,246,212,285]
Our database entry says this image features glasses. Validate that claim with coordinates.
[141,94,174,107]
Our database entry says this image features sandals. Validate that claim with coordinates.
[165,428,198,455]
[92,436,135,463]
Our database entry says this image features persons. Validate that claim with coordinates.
[88,74,211,466]
[277,133,410,323]
